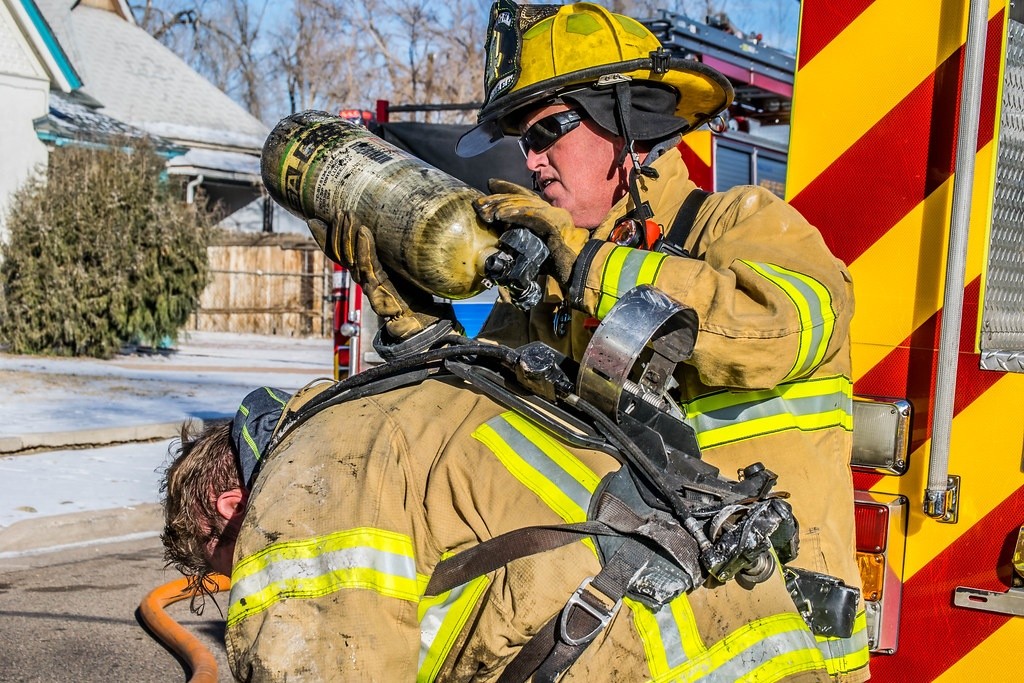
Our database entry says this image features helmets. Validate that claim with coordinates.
[454,0,733,159]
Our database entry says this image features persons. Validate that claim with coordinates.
[159,359,836,683]
[303,0,873,683]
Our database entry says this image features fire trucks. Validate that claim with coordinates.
[321,1,1024,683]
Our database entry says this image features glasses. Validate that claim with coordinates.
[518,109,590,159]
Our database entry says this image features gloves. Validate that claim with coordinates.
[306,209,444,338]
[472,178,589,288]
